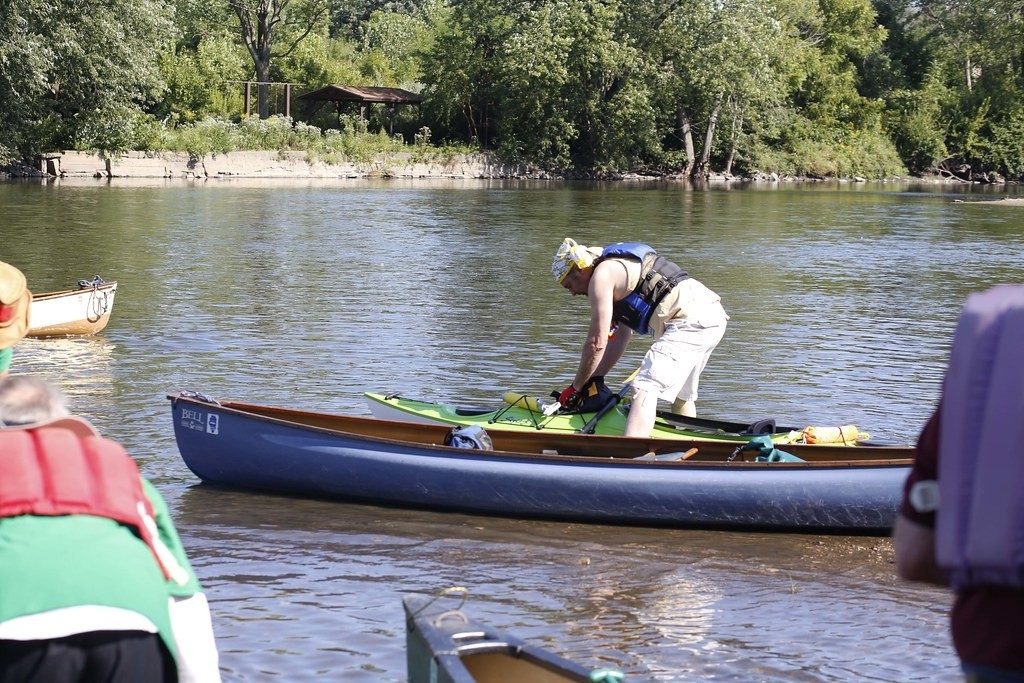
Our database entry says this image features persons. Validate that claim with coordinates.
[2,376,224,683]
[896,283,1024,683]
[557,237,731,439]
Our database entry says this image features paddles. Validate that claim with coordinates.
[579,367,641,434]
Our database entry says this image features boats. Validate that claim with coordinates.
[390,593,627,682]
[166,388,920,535]
[24,275,118,340]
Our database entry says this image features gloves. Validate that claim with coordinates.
[558,383,585,411]
